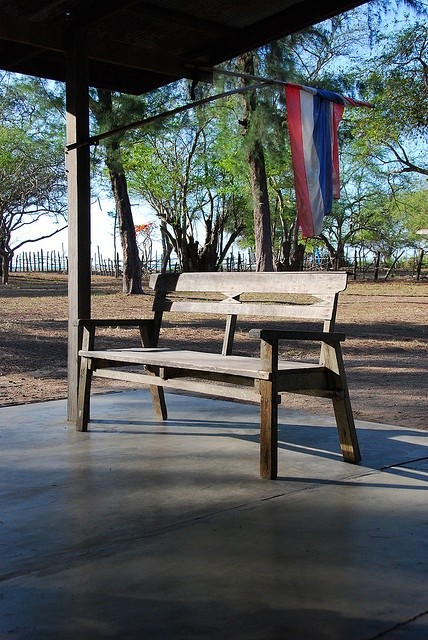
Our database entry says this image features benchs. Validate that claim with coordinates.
[72,269,362,481]
